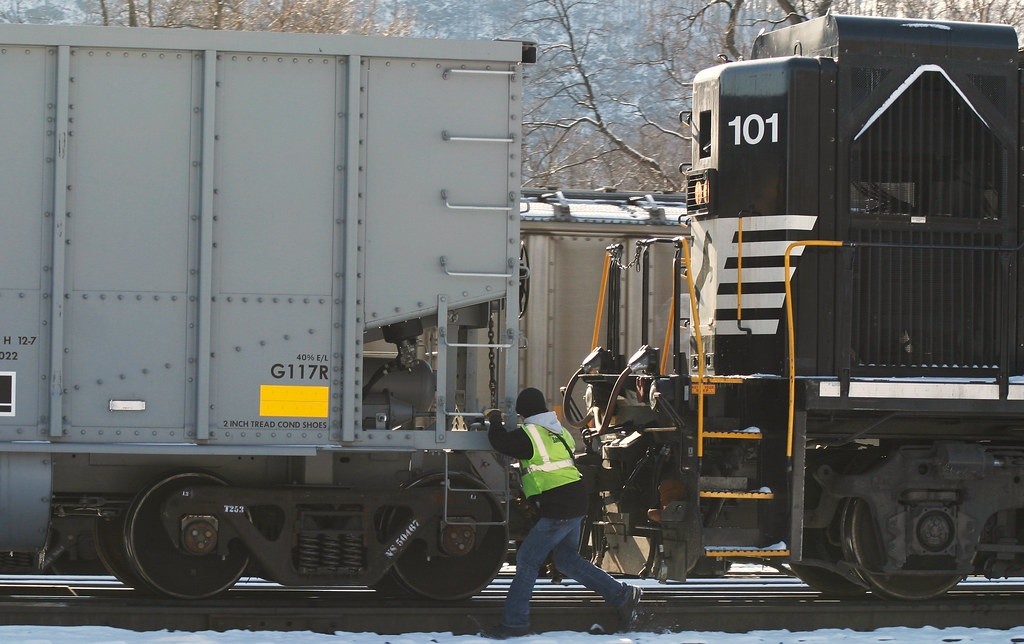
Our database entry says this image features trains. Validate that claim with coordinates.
[561,9,1024,603]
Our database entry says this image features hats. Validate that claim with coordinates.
[515,388,548,416]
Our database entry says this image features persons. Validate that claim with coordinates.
[481,387,644,640]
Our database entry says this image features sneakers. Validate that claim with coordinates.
[479,622,532,640]
[619,586,642,631]
